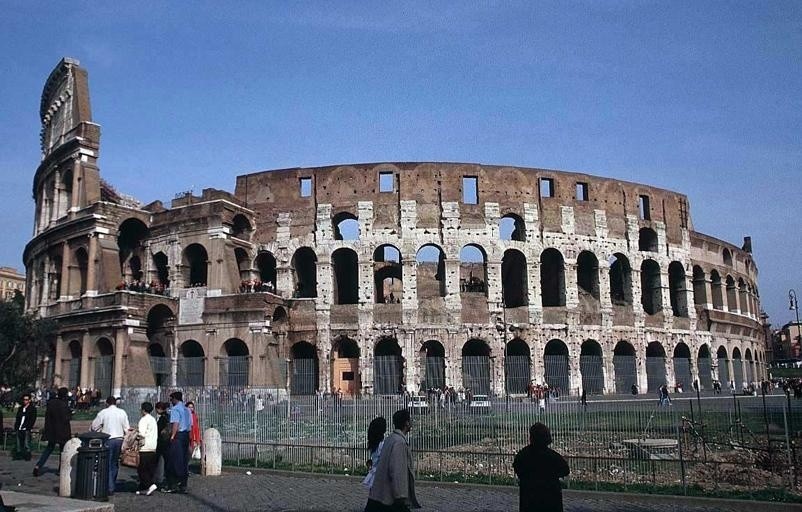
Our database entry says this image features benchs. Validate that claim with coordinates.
[510,393,528,403]
[240,387,287,408]
[406,392,492,414]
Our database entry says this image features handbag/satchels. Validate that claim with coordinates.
[120,441,140,468]
[191,446,201,460]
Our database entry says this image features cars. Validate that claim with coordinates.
[408,395,430,415]
[469,394,493,415]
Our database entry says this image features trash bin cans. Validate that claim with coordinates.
[77,433,111,502]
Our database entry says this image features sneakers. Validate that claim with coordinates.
[146,484,157,495]
[136,490,148,495]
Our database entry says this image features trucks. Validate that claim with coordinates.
[226,389,288,413]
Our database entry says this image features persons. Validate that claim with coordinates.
[34,387,72,477]
[676,382,684,393]
[631,383,640,395]
[117,279,164,295]
[241,278,273,294]
[13,394,38,462]
[136,391,201,496]
[211,386,263,416]
[713,378,802,399]
[527,381,561,414]
[362,409,421,512]
[581,389,588,411]
[461,280,485,293]
[91,395,130,495]
[659,385,672,406]
[512,423,570,512]
[390,292,394,303]
[314,387,343,411]
[69,386,101,409]
[428,386,473,408]
[33,385,58,405]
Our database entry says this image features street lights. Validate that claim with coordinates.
[495,322,515,413]
[789,289,802,358]
